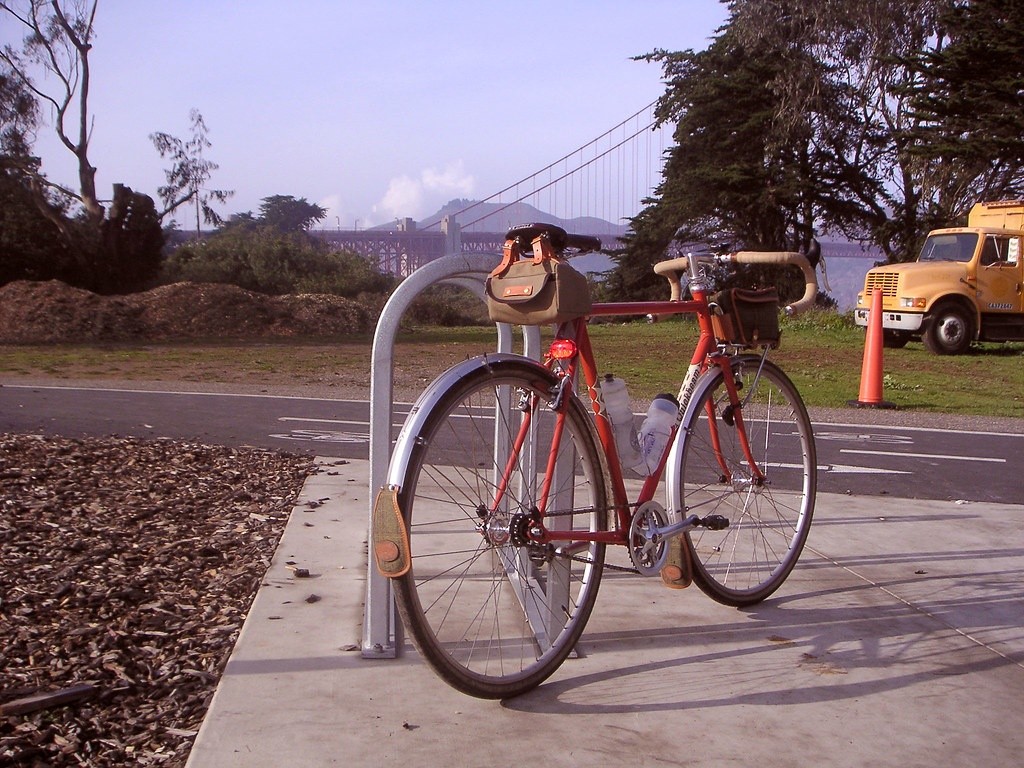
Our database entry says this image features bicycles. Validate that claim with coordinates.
[372,222,818,700]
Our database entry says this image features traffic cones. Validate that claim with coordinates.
[844,289,898,409]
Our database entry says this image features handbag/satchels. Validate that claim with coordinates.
[486,234,591,325]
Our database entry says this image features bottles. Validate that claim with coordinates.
[631,392,680,477]
[600,373,643,470]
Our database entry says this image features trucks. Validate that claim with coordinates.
[853,195,1024,357]
